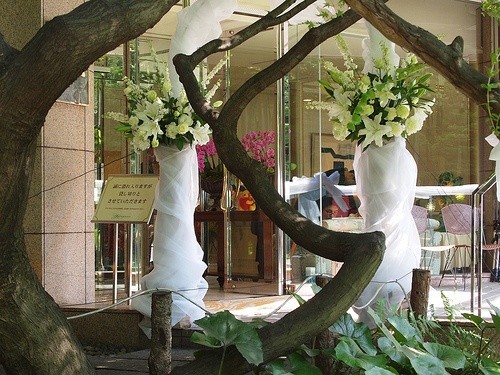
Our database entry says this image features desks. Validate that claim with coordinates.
[193,210,274,289]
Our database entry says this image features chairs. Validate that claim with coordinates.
[440,204,500,292]
[408,205,456,287]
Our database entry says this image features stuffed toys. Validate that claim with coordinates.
[236,185,256,211]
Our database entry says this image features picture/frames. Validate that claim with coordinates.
[311,133,357,179]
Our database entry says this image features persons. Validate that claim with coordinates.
[315,168,363,221]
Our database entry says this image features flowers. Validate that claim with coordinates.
[196,139,227,209]
[240,130,276,172]
[303,0,445,330]
[109,0,243,339]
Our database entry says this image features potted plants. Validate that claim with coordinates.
[438,171,457,186]
